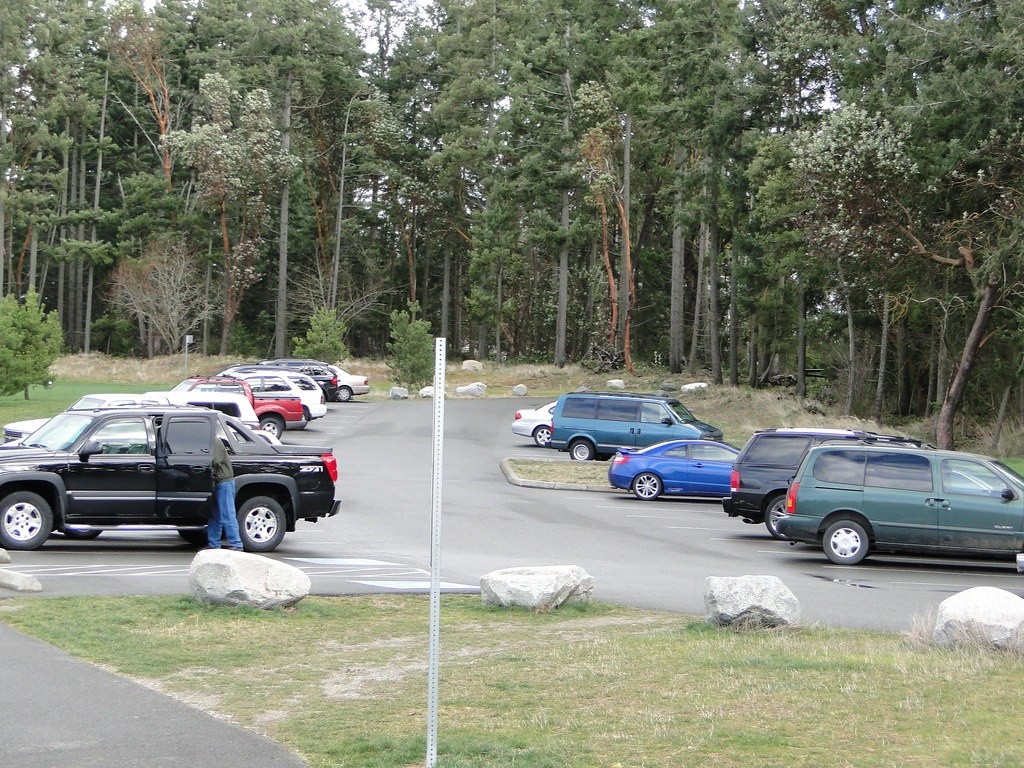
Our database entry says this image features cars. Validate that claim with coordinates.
[328,364,370,402]
[243,371,329,420]
[256,357,339,404]
[2,392,184,443]
[512,400,558,448]
[608,438,742,503]
[146,391,261,429]
[215,364,303,377]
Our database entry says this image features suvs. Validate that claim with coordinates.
[722,428,938,542]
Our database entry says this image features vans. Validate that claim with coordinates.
[549,390,725,463]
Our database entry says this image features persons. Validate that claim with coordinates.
[196,436,244,552]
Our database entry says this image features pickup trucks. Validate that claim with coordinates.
[169,375,303,439]
[0,400,343,554]
[778,444,1024,566]
